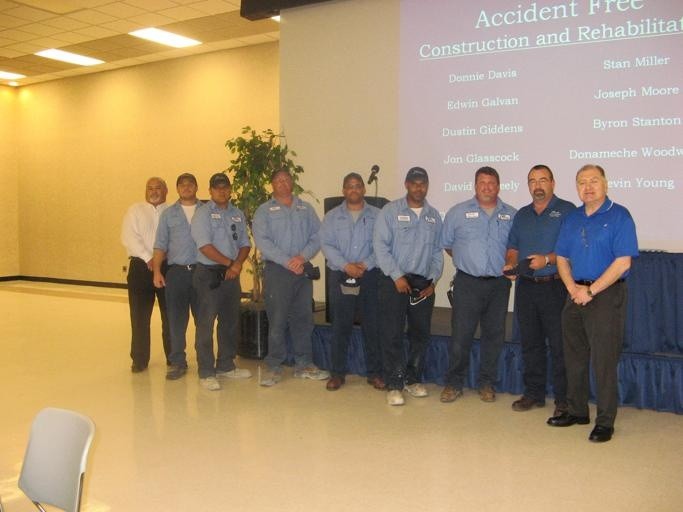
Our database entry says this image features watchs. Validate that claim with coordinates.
[544,254,551,268]
[587,285,595,299]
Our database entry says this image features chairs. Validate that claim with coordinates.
[17,407,95,512]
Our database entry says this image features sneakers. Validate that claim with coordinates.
[404,382,428,396]
[200,376,220,390]
[294,367,329,379]
[216,368,251,378]
[260,370,282,386]
[478,384,496,402]
[441,385,463,402]
[388,388,404,405]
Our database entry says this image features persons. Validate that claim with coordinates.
[252,168,331,387]
[190,173,251,390]
[438,166,518,403]
[317,172,385,391]
[546,164,640,443]
[372,166,444,405]
[121,177,172,373]
[502,165,577,418]
[154,173,207,380]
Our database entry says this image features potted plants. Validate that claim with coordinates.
[217,125,320,360]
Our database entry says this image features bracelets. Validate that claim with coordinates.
[228,260,234,269]
[431,283,436,289]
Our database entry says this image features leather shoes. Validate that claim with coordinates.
[132,359,148,371]
[589,425,614,442]
[547,412,590,426]
[513,396,545,411]
[166,360,188,379]
[327,375,345,390]
[367,375,385,389]
[554,402,568,416]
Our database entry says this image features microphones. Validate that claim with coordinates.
[368,164,379,184]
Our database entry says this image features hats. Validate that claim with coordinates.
[210,173,230,188]
[406,167,428,180]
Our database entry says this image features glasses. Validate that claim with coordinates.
[231,224,238,240]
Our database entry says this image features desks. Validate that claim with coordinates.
[511,252,683,358]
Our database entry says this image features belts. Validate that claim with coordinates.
[575,280,624,287]
[520,273,562,283]
[174,264,193,271]
